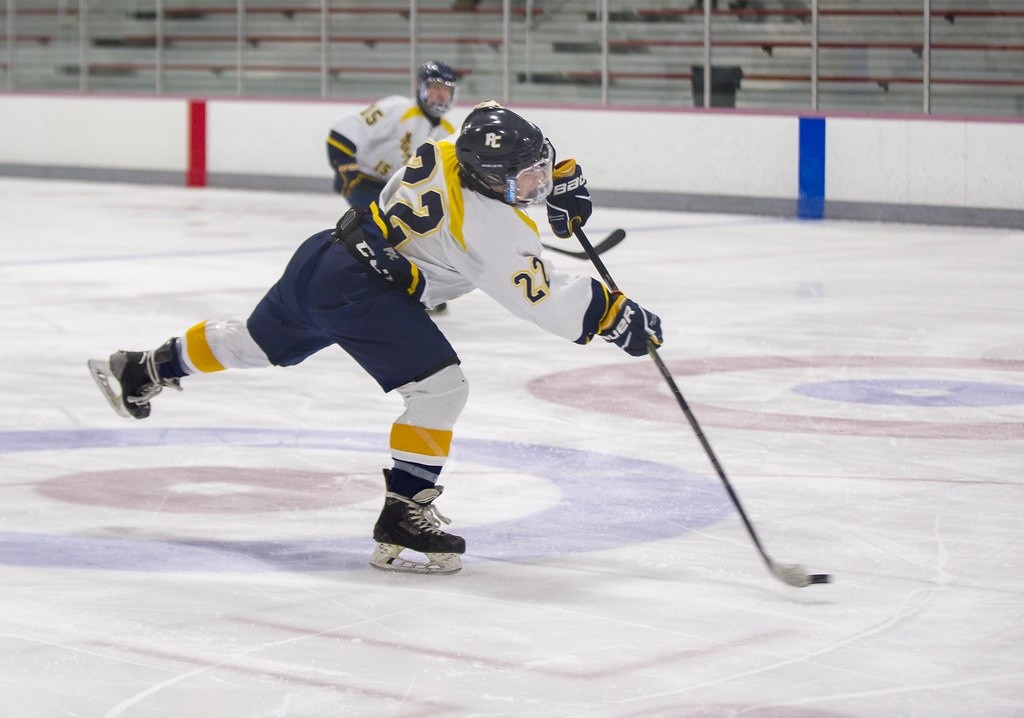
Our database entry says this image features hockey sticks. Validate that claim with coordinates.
[540,227,626,261]
[571,218,812,589]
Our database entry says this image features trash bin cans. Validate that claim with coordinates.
[690,64,744,108]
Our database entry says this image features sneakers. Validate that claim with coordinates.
[427,302,447,318]
[88,337,183,420]
[369,468,465,573]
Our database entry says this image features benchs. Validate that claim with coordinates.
[0,0,1024,117]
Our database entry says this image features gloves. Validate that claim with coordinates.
[334,171,389,214]
[596,290,663,357]
[547,159,593,239]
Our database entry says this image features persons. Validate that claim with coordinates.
[88,97,662,575]
[326,57,456,313]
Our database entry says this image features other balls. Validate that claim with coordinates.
[807,573,833,585]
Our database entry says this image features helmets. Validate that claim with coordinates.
[418,61,456,87]
[456,99,545,186]
